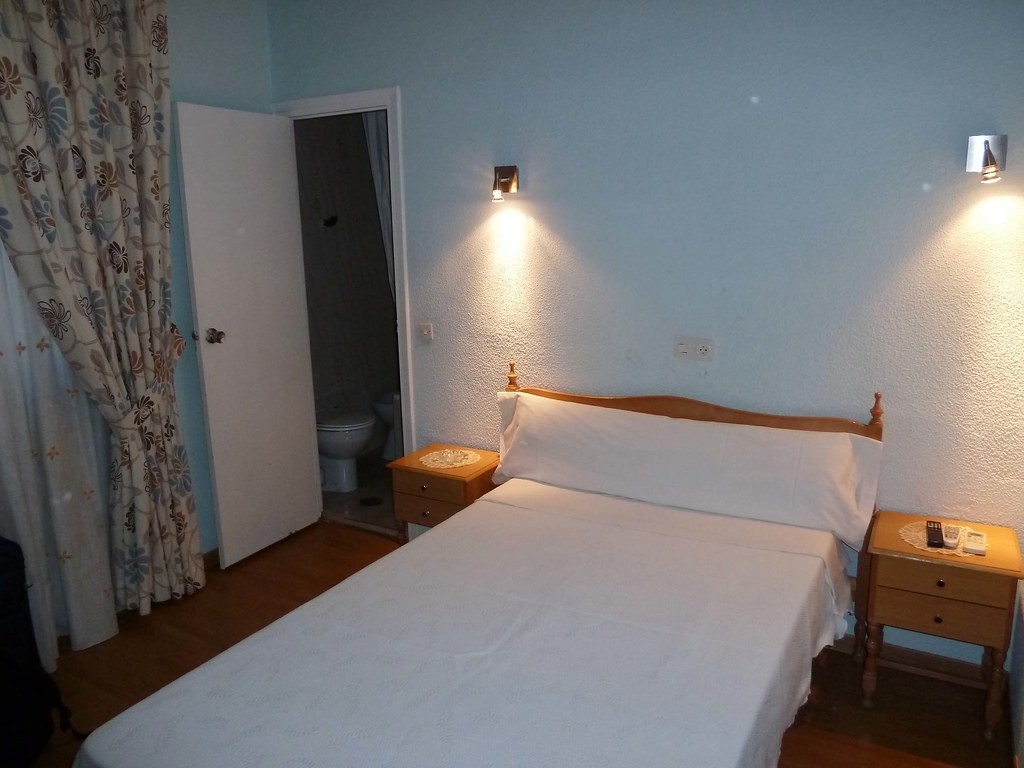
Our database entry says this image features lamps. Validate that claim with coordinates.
[966,136,1008,183]
[491,165,519,202]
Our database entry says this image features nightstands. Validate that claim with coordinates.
[862,511,1024,741]
[386,444,501,546]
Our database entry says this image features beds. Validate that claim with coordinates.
[72,362,882,768]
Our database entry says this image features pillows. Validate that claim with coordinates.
[491,392,882,551]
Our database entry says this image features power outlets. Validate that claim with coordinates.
[674,338,716,360]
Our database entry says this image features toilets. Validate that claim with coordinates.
[315,405,376,495]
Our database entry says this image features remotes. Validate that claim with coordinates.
[926,520,944,548]
[944,526,961,548]
[963,530,986,556]
